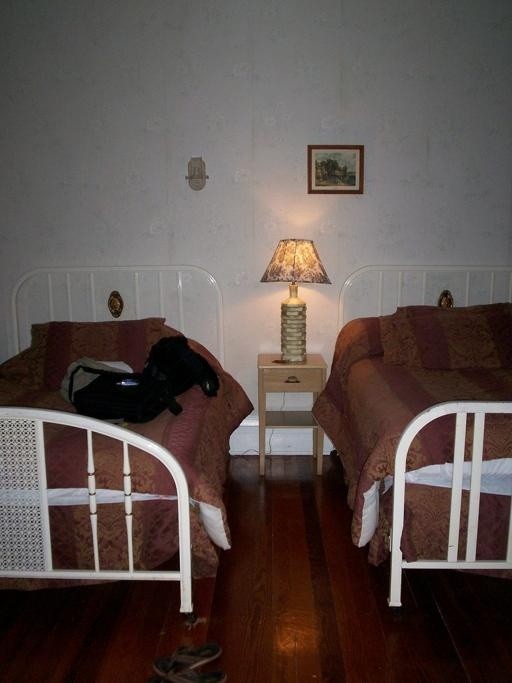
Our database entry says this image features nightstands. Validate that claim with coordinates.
[257,353,328,476]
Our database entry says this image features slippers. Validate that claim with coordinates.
[153,644,221,676]
[146,672,226,683]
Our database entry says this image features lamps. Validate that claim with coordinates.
[260,239,331,364]
[185,157,210,191]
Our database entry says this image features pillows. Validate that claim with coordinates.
[30,317,166,394]
[379,303,512,370]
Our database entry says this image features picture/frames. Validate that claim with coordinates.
[307,143,365,195]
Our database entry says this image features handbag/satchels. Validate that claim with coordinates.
[69,366,182,423]
[60,357,129,402]
[143,335,219,398]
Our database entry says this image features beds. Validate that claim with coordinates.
[2,267,254,624]
[310,265,512,620]
